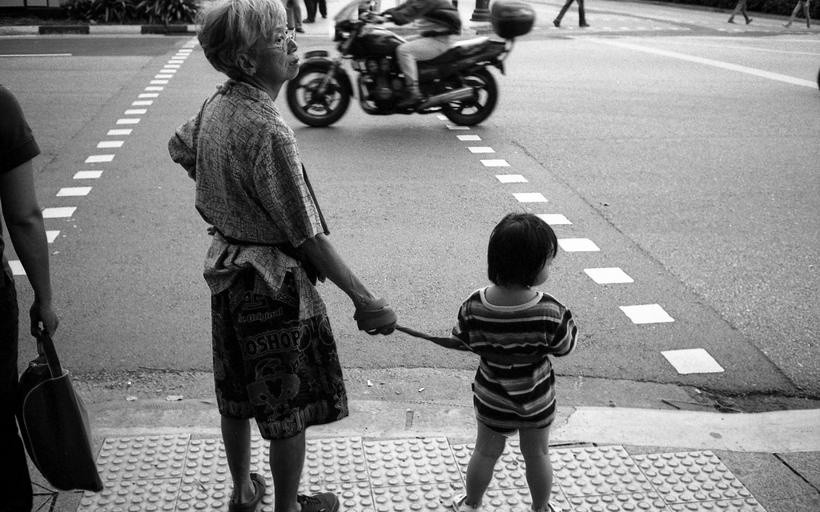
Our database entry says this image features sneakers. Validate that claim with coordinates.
[398,92,425,108]
[451,494,479,512]
[229,473,267,512]
[297,492,339,512]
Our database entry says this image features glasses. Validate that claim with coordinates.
[254,27,296,53]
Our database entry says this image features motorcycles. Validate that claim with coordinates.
[287,0,536,127]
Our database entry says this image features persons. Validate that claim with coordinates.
[283,0,328,33]
[167,0,397,512]
[727,0,811,29]
[450,213,579,511]
[1,83,60,512]
[552,0,590,28]
[360,0,462,105]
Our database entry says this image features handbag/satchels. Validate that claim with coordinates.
[16,332,104,492]
